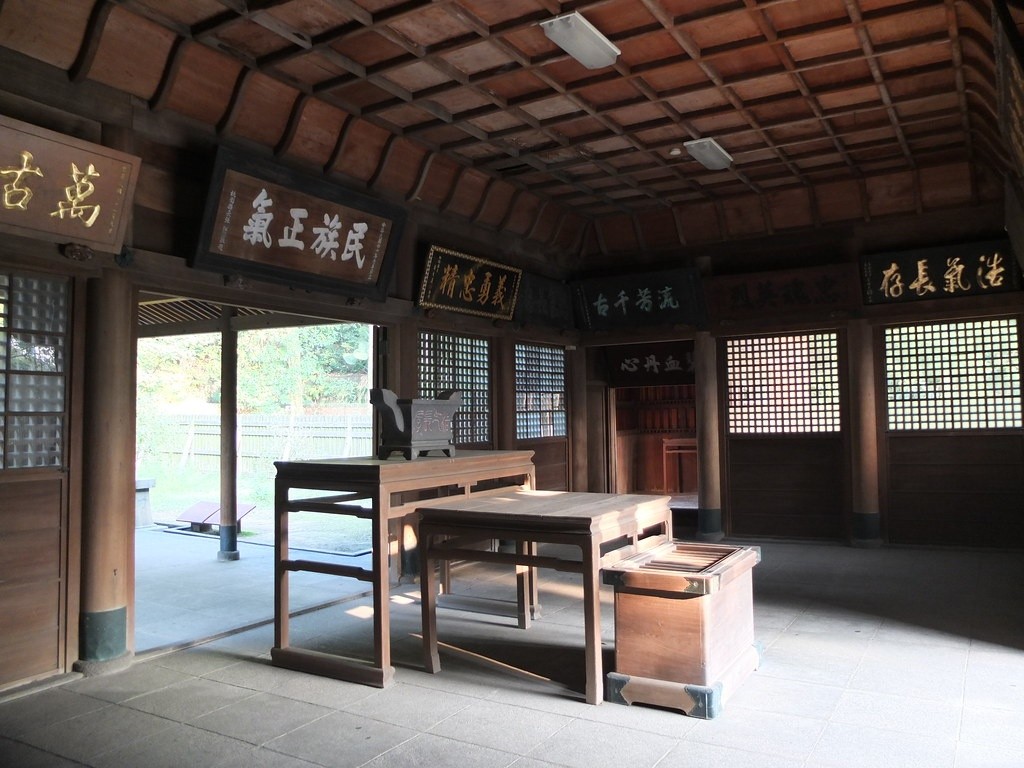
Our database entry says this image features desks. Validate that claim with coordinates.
[414,490,672,706]
[273,449,538,688]
[662,438,697,495]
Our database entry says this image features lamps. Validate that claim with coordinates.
[538,11,622,70]
[683,137,733,170]
